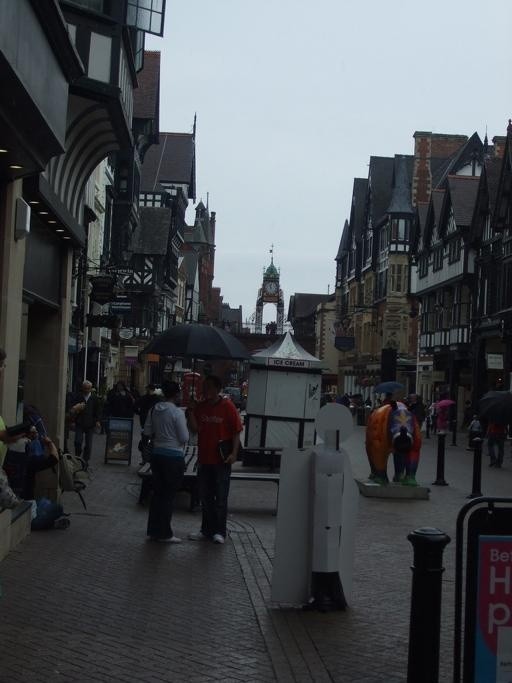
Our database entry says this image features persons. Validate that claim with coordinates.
[188,376,244,544]
[407,393,426,430]
[438,408,447,436]
[104,380,138,419]
[428,396,438,435]
[71,379,103,463]
[4,424,71,530]
[382,391,392,404]
[139,380,189,543]
[134,383,157,463]
[467,414,481,433]
[487,420,507,467]
[0,346,20,509]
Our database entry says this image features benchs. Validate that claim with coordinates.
[137,445,201,510]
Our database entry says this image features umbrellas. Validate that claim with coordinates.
[372,379,402,395]
[470,389,512,417]
[433,398,456,409]
[142,319,248,401]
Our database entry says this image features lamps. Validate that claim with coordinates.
[73,268,115,306]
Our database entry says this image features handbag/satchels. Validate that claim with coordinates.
[218,438,242,459]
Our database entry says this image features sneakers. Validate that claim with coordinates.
[213,533,225,543]
[188,531,207,541]
[488,462,502,468]
[150,535,181,542]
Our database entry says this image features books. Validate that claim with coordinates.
[218,439,246,461]
